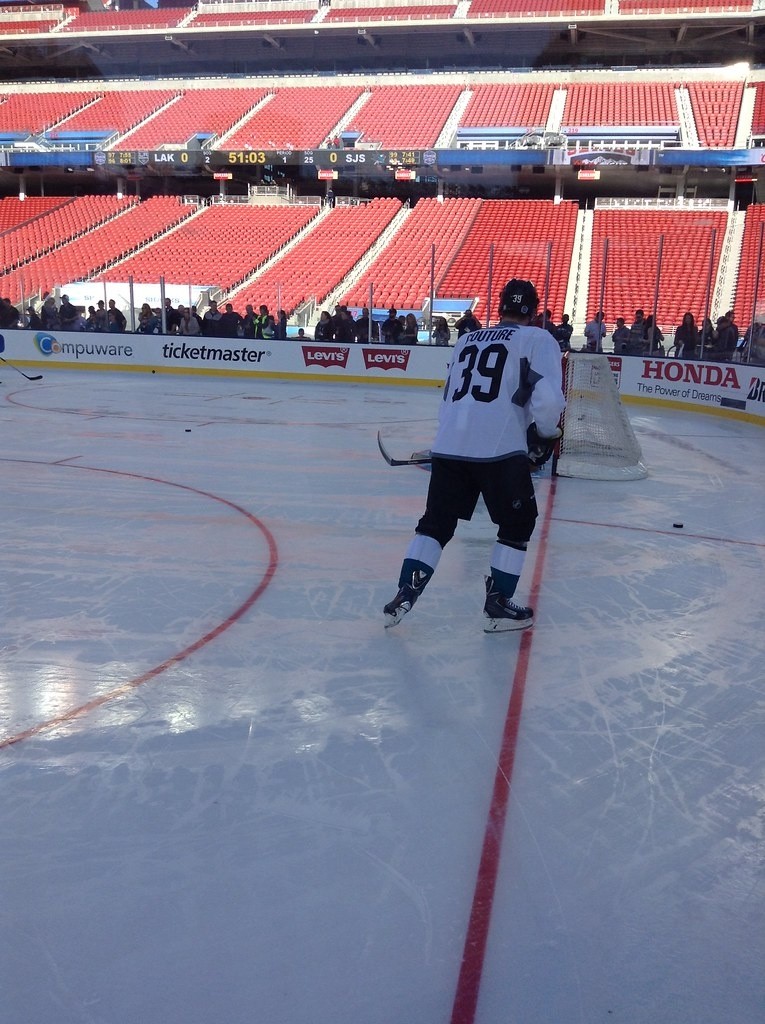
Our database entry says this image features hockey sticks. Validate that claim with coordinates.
[377,429,432,467]
[0,357,43,381]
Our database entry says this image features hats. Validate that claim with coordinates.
[26,306,34,312]
[387,308,397,314]
[97,300,104,304]
[59,295,69,301]
[715,315,728,324]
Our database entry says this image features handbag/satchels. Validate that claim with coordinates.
[652,339,665,357]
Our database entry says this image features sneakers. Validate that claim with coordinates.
[383,569,431,630]
[479,574,534,633]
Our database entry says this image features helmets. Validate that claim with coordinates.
[496,279,540,319]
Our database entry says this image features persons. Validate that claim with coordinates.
[268,310,291,338]
[326,137,332,144]
[136,298,202,337]
[25,295,127,332]
[432,317,451,346]
[383,278,565,635]
[584,312,607,353]
[314,305,379,343]
[454,310,482,340]
[533,309,573,351]
[202,298,273,339]
[380,308,418,345]
[611,309,665,356]
[0,297,20,328]
[296,328,308,338]
[333,136,340,149]
[672,311,739,363]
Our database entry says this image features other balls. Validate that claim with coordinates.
[438,385,441,388]
[672,523,684,529]
[185,429,192,432]
[152,370,156,374]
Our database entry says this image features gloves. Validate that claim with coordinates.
[525,422,563,471]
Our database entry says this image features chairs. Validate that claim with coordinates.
[0,0,765,151]
[0,195,579,323]
[585,210,728,325]
[732,204,765,326]
